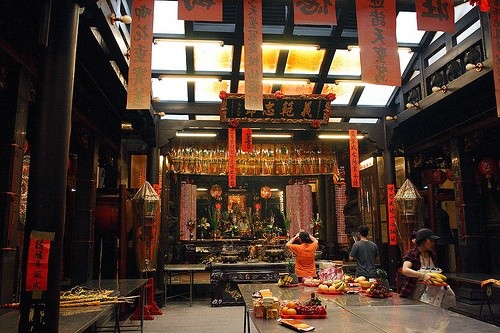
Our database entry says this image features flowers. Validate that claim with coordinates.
[198,217,210,234]
[187,219,196,233]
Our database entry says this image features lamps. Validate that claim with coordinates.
[176,131,218,137]
[153,38,225,47]
[263,78,310,85]
[386,115,400,121]
[111,13,132,25]
[261,42,320,51]
[152,96,159,102]
[334,80,370,86]
[158,74,222,82]
[432,84,447,93]
[465,62,483,71]
[406,102,419,110]
[252,132,294,138]
[348,46,412,53]
[154,111,165,117]
[317,132,365,139]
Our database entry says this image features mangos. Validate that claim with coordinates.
[334,280,346,290]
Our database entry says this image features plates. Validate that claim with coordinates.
[420,270,442,273]
[280,312,327,319]
[281,284,299,287]
[359,289,393,298]
[417,281,447,286]
[318,289,343,294]
[303,282,319,287]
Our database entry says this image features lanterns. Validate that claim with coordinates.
[473,157,500,193]
[430,169,456,184]
[261,186,271,199]
[210,185,222,197]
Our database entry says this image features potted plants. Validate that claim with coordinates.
[208,205,217,241]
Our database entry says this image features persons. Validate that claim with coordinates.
[286,230,319,283]
[400,228,440,300]
[349,225,381,280]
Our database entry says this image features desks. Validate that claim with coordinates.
[0,279,148,333]
[163,262,500,332]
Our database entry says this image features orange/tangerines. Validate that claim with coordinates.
[282,301,297,315]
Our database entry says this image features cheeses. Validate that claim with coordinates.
[359,281,370,287]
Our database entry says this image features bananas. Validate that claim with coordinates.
[425,273,447,283]
[281,275,293,284]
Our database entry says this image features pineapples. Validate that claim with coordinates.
[285,261,298,283]
[378,269,389,290]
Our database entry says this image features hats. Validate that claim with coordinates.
[416,228,440,241]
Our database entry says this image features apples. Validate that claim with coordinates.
[295,302,327,315]
[318,281,335,292]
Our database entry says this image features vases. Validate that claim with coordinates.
[190,233,194,240]
[201,234,204,241]
